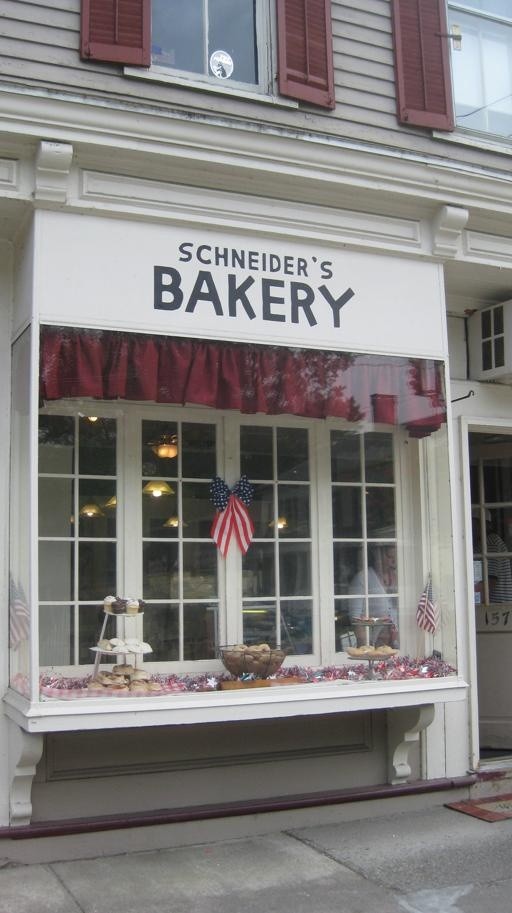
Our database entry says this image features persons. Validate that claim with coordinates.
[469,504,511,601]
[348,541,399,649]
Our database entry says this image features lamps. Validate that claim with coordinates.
[79,486,104,522]
[268,511,288,529]
[143,420,190,527]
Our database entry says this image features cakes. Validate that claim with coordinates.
[86,637,152,696]
[103,597,143,618]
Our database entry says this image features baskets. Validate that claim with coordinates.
[217,644,286,680]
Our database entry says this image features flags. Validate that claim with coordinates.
[415,574,442,634]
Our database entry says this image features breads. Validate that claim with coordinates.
[219,641,285,665]
[345,645,396,659]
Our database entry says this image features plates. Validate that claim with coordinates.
[351,621,395,627]
[103,609,146,619]
[90,646,153,656]
[347,656,390,662]
